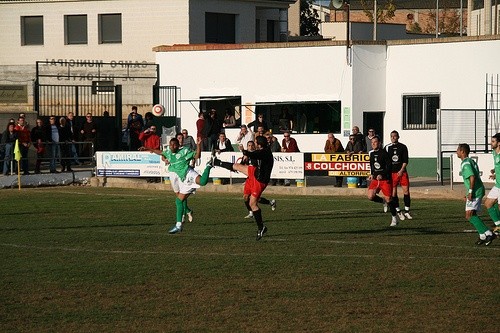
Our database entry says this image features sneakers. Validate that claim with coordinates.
[210,149,217,167]
[244,211,254,218]
[402,210,412,219]
[397,210,405,220]
[482,232,497,247]
[493,226,500,235]
[382,202,388,213]
[206,160,215,168]
[256,225,267,241]
[270,199,276,211]
[390,215,397,226]
[168,225,183,233]
[475,235,485,246]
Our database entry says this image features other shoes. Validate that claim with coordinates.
[334,183,343,187]
[271,179,291,186]
[356,180,368,187]
[147,177,162,183]
[182,209,193,223]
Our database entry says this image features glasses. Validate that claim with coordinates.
[350,138,355,140]
[266,136,270,139]
[284,135,290,138]
[87,116,92,118]
[369,130,374,133]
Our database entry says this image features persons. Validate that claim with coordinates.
[182,129,197,170]
[0,111,100,176]
[126,106,144,151]
[365,128,382,153]
[352,126,368,153]
[162,132,195,223]
[144,112,156,129]
[324,133,346,187]
[196,105,240,152]
[367,136,398,226]
[213,135,276,240]
[456,143,497,245]
[212,132,235,185]
[254,124,300,187]
[138,138,215,234]
[247,113,268,135]
[483,133,500,239]
[383,130,413,220]
[344,135,368,188]
[137,125,160,151]
[236,124,255,151]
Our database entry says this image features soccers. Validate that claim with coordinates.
[153,104,165,117]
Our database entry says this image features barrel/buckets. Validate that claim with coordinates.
[297,179,305,188]
[347,176,359,188]
[213,177,223,185]
[164,177,171,185]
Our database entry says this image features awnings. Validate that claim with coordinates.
[178,96,240,114]
[240,101,341,116]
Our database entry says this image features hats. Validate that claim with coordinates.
[130,112,137,116]
[199,108,216,116]
[50,117,55,120]
